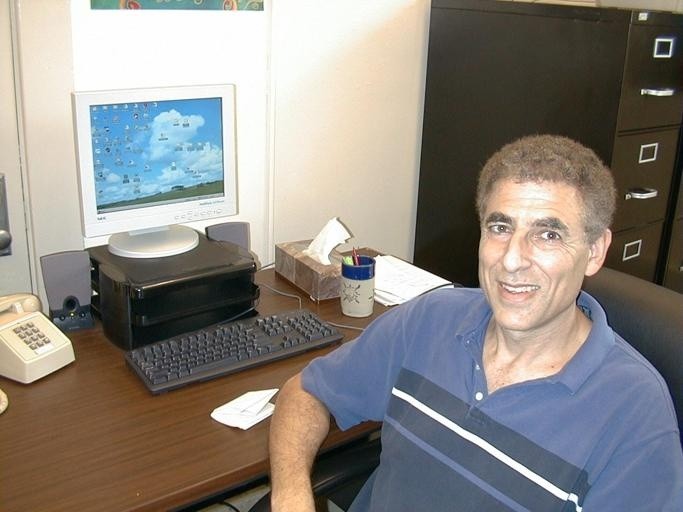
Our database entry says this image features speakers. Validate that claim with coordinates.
[40,250,95,332]
[205,222,248,251]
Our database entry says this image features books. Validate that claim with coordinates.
[374,252,455,308]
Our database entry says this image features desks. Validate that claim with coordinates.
[0,248,464,512]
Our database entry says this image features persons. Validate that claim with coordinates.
[265,132,682,511]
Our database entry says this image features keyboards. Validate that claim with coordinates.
[124,308,345,395]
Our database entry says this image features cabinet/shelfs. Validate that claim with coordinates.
[411,0,683,290]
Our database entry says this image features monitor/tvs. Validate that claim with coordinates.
[71,84,237,260]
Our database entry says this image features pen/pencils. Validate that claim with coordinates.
[343,246,360,266]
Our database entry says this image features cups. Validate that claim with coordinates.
[340,255,375,318]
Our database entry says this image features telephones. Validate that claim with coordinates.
[0,293,75,385]
